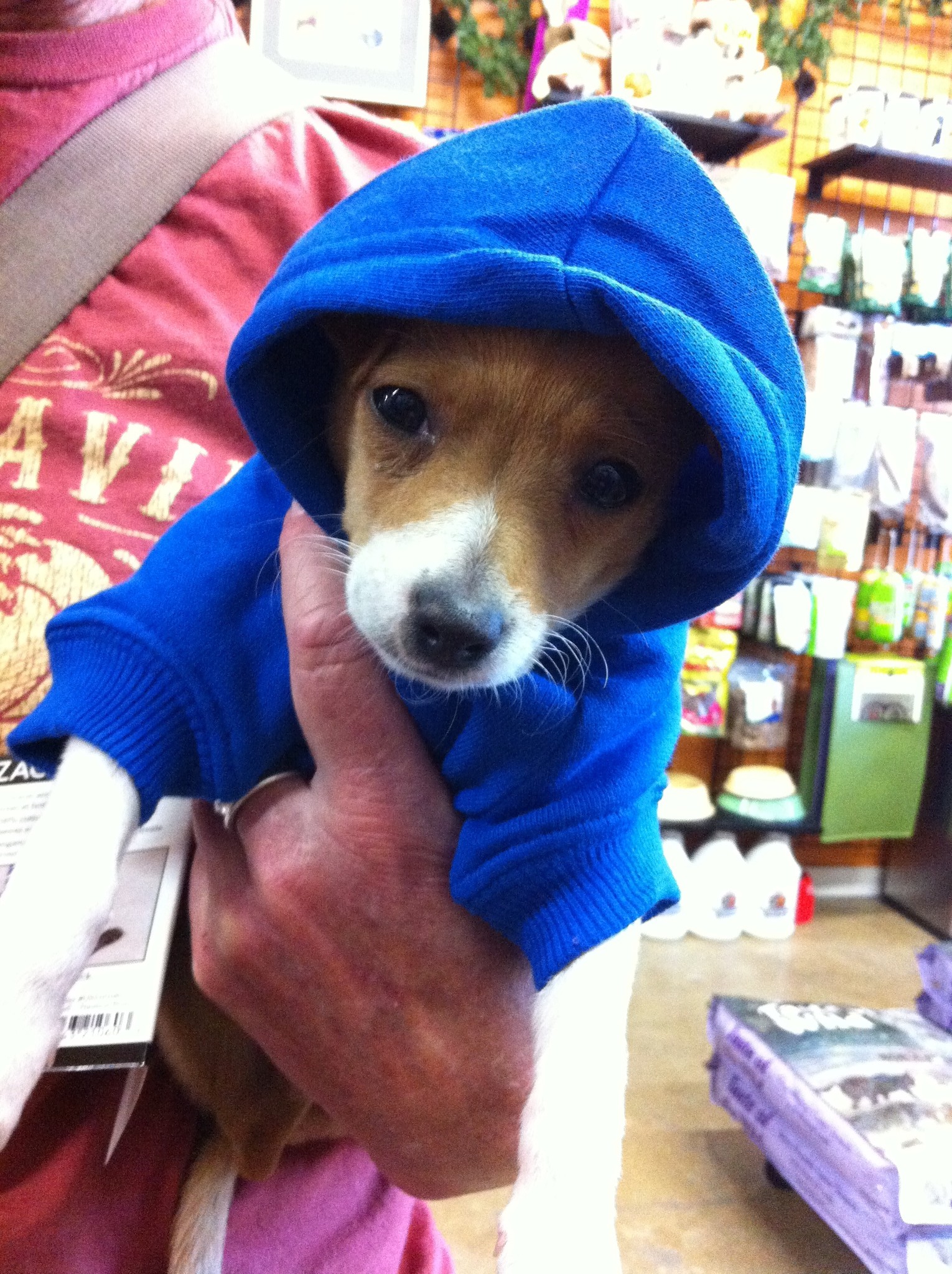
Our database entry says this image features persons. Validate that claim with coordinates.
[0,1,537,1274]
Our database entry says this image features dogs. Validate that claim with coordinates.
[0,96,806,1274]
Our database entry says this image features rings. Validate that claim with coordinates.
[214,768,299,830]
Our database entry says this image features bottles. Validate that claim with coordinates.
[744,832,802,938]
[688,830,749,942]
[637,827,696,943]
